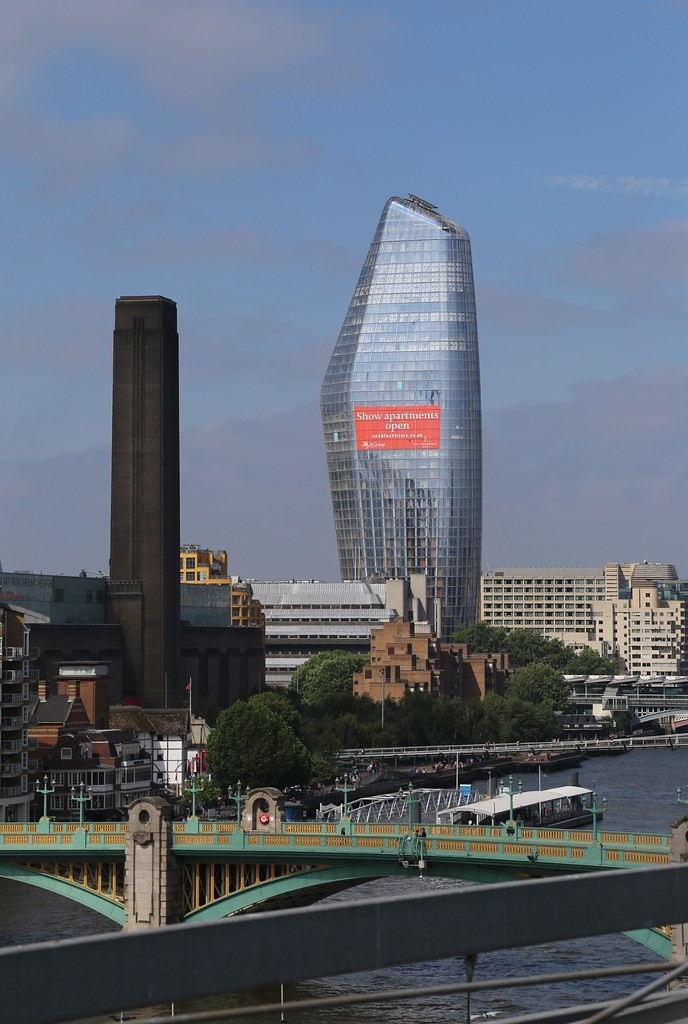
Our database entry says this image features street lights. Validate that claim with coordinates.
[70,781,93,833]
[581,790,608,844]
[334,772,357,820]
[500,774,524,829]
[184,773,204,823]
[35,775,57,822]
[398,781,423,837]
[227,780,250,834]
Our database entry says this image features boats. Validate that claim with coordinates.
[435,765,604,829]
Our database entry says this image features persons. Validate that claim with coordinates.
[484,729,627,757]
[340,828,346,835]
[421,828,426,837]
[415,830,422,837]
[282,756,484,796]
[185,795,246,822]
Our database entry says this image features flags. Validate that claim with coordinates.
[175,770,177,780]
[185,677,191,690]
